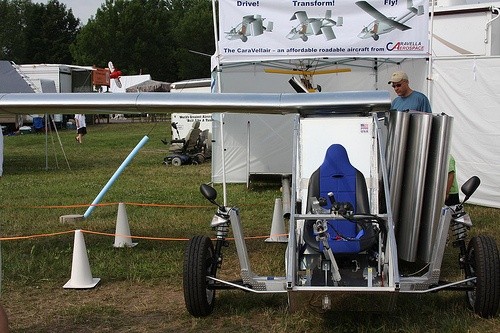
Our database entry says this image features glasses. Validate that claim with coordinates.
[393,81,405,88]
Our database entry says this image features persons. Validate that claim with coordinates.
[387,71,433,113]
[74,113,87,145]
[444,153,464,244]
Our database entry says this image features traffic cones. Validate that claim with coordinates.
[62,230,102,289]
[112,202,138,248]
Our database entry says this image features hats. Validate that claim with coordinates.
[388,71,408,84]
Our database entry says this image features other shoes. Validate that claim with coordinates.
[75,137,79,143]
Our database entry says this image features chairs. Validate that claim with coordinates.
[302,143,378,255]
[168,121,201,152]
